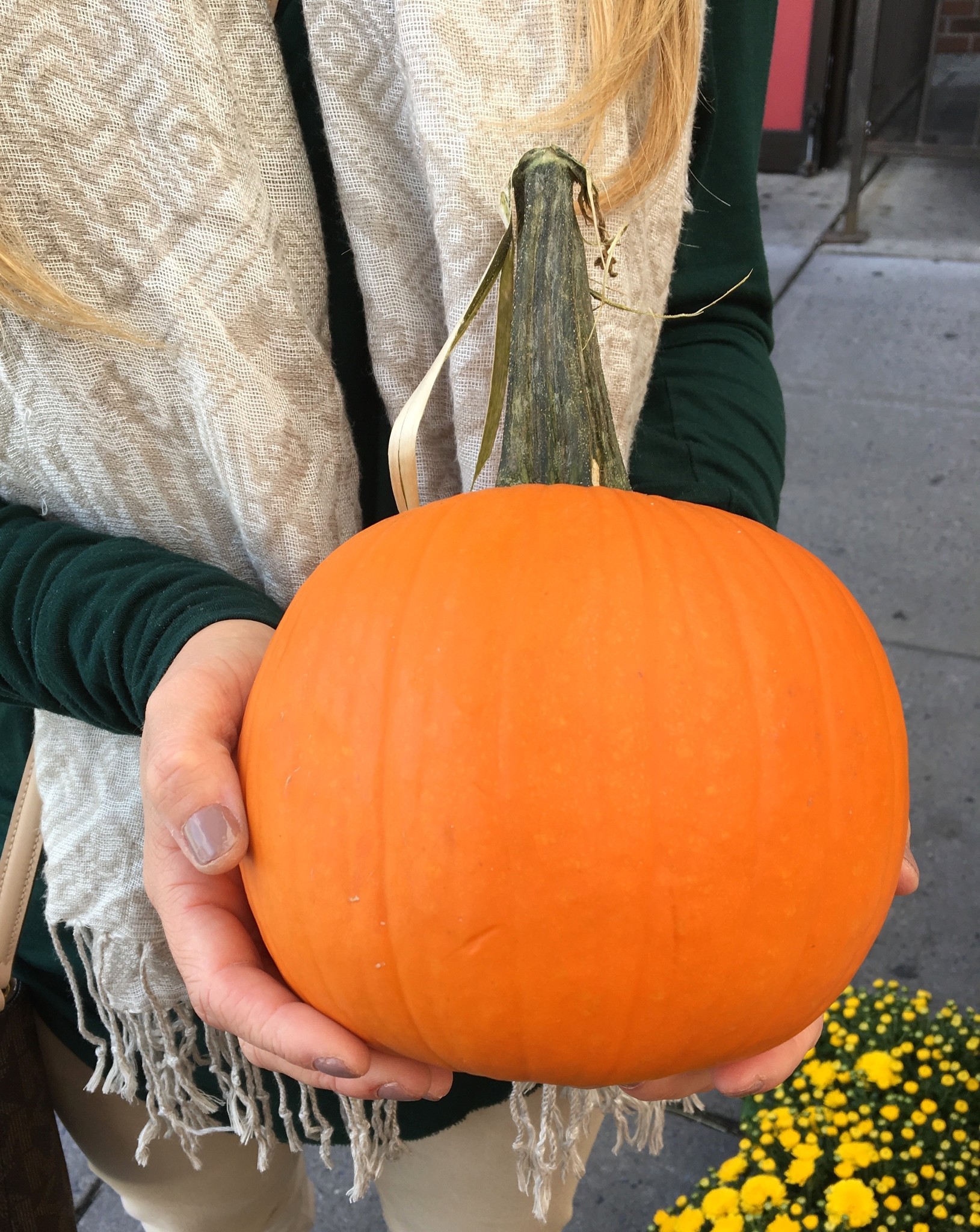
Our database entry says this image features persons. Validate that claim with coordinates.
[0,0,915,1232]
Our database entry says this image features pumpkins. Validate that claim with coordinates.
[235,147,908,1087]
